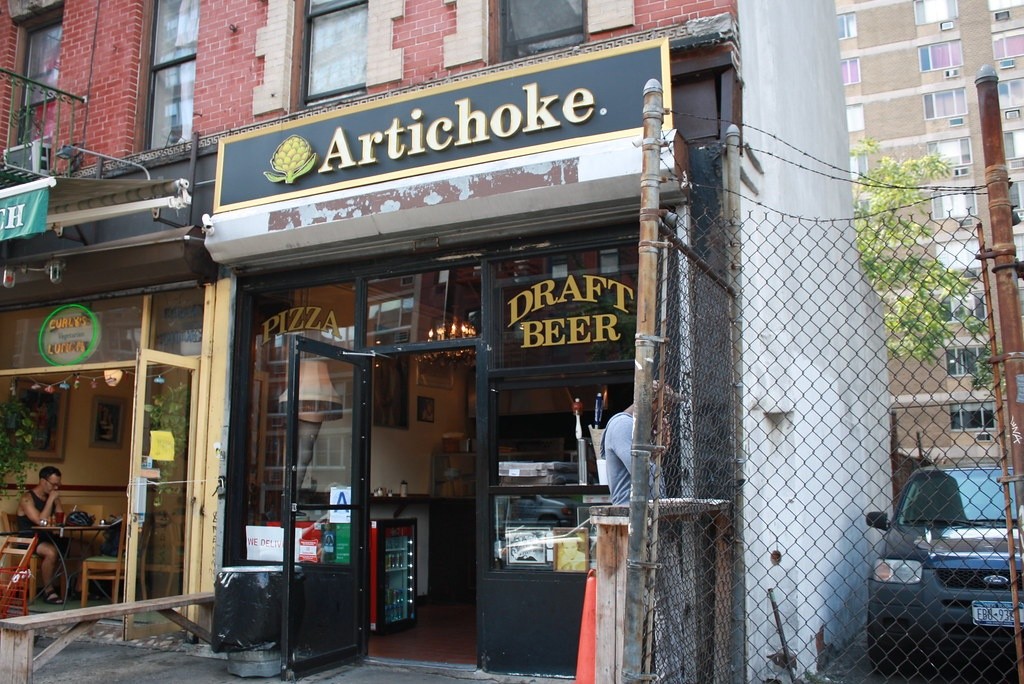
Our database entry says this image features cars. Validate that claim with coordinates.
[508,496,572,524]
[866,465,1023,675]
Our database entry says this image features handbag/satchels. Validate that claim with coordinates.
[100,519,120,557]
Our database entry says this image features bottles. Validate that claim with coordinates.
[400,480,407,497]
[375,486,392,498]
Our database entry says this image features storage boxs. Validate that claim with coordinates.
[299,520,351,563]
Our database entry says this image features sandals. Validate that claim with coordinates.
[43,590,63,604]
[73,590,100,601]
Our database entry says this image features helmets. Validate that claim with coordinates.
[65,511,92,526]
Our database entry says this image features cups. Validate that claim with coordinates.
[55,512,64,526]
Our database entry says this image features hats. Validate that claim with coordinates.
[651,380,684,402]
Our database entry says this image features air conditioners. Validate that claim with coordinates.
[974,432,992,441]
[941,9,1024,229]
[3,142,53,175]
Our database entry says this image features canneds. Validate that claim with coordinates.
[386,587,403,622]
[385,551,404,569]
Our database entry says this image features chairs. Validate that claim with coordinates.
[0,504,185,608]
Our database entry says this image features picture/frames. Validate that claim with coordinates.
[89,395,128,449]
[415,365,455,424]
[10,377,70,464]
[555,527,590,573]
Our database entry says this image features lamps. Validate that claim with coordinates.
[551,247,621,279]
[415,270,477,366]
[278,289,344,423]
[56,145,150,180]
[0,257,66,290]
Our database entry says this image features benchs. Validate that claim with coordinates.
[0,592,216,684]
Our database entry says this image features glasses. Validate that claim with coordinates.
[40,477,62,489]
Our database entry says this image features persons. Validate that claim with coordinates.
[14,465,101,605]
[595,367,683,508]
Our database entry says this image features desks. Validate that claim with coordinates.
[28,516,123,611]
